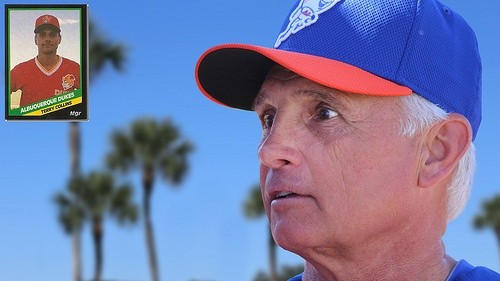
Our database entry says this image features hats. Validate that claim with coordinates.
[190,1,483,140]
[34,13,61,32]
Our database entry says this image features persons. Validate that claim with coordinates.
[194,1,496,281]
[10,15,81,106]
